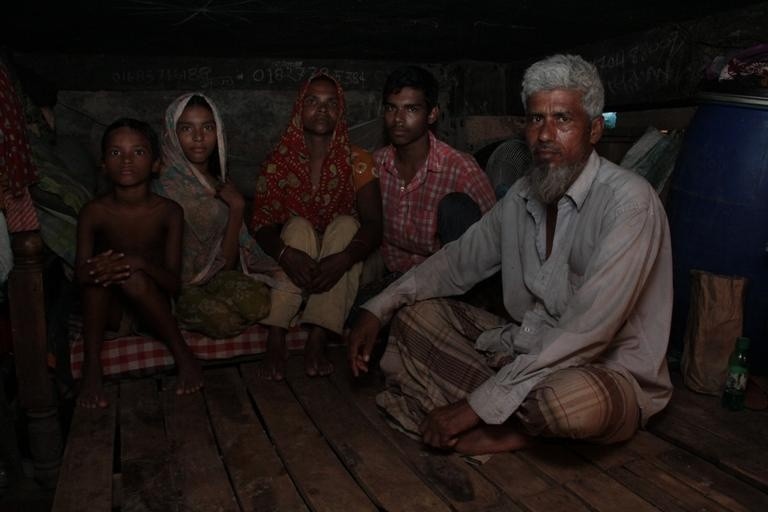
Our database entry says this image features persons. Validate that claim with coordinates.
[149,92,272,339]
[347,54,675,458]
[360,65,496,385]
[77,118,204,410]
[248,73,383,382]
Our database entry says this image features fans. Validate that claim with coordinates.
[485,139,534,200]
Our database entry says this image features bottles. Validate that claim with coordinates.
[721,336,752,410]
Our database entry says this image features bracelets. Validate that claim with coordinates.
[278,246,288,264]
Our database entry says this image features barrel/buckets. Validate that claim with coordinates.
[666,92,768,378]
